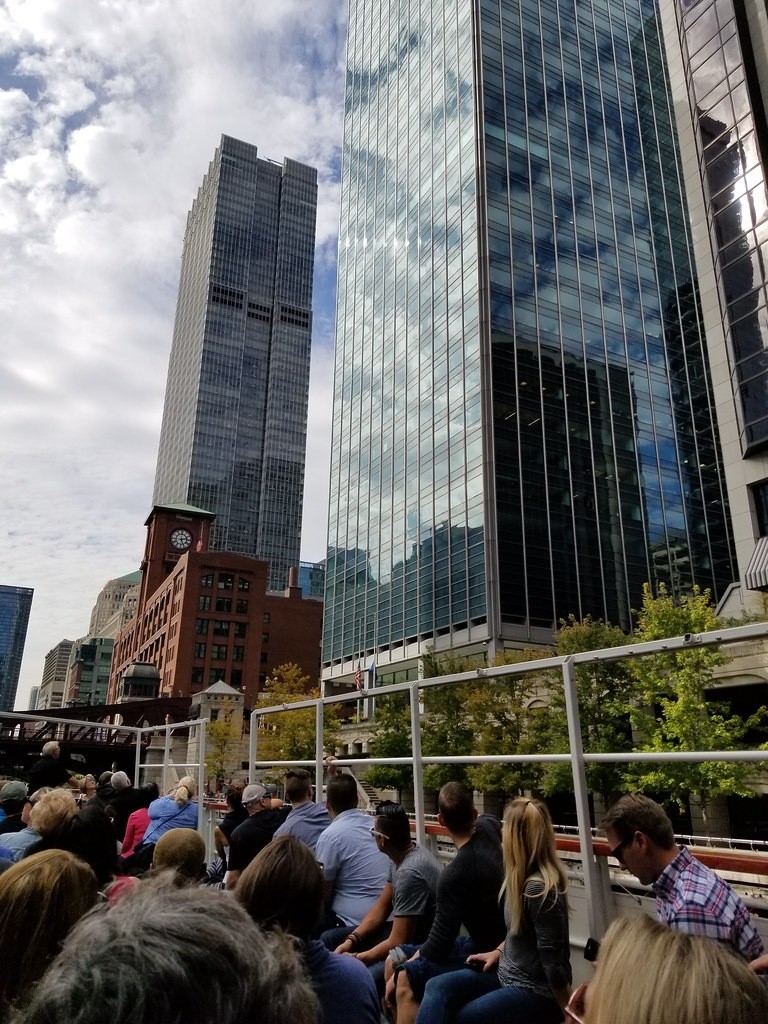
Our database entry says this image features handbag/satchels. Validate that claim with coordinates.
[133,840,144,853]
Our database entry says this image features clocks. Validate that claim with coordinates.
[170,527,193,550]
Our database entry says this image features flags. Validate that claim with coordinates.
[354,661,361,689]
[369,661,374,672]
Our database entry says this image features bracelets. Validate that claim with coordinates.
[352,952,357,957]
[496,948,503,953]
[345,932,362,944]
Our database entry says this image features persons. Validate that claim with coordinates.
[316,773,396,926]
[385,779,506,1024]
[145,826,224,890]
[23,889,320,1024]
[594,791,764,967]
[583,914,768,1024]
[232,835,384,1024]
[0,849,101,1024]
[0,770,159,902]
[215,782,292,889]
[367,800,375,810]
[142,776,198,844]
[26,741,80,796]
[333,800,443,1010]
[273,755,338,851]
[415,794,573,1024]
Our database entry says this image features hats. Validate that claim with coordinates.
[0,781,27,804]
[241,783,278,808]
[98,772,114,784]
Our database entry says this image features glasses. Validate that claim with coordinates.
[369,827,390,840]
[263,793,272,799]
[612,833,635,864]
[565,982,589,1024]
